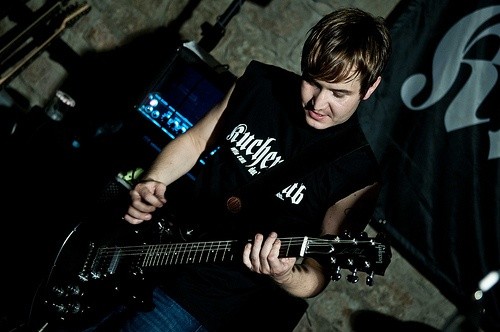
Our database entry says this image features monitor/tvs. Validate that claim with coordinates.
[132,45,237,169]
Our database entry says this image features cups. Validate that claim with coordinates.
[47,90,76,121]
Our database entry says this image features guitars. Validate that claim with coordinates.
[44,204,393,332]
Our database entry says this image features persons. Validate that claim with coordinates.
[89,8,390,332]
